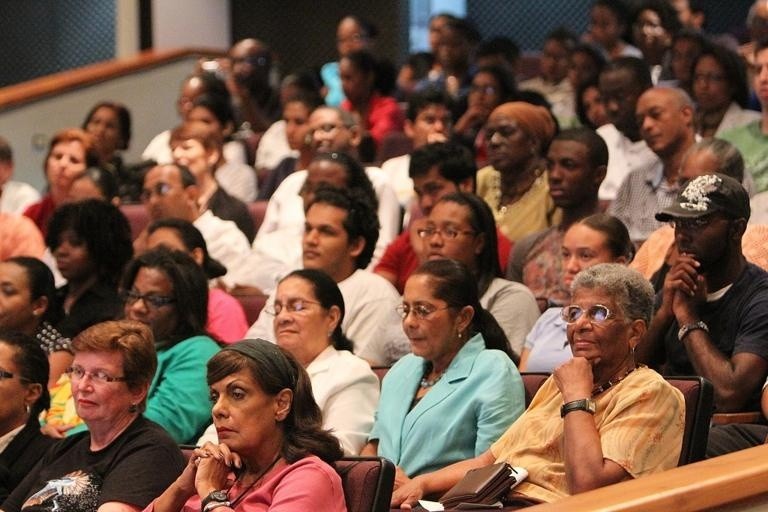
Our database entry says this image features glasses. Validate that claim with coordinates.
[394,303,447,320]
[65,363,124,385]
[264,298,317,317]
[666,217,707,231]
[558,303,621,324]
[0,369,32,384]
[139,182,180,202]
[416,228,467,242]
[118,285,172,311]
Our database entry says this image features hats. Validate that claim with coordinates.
[655,172,751,224]
[489,101,556,150]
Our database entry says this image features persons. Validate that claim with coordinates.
[0,0,767,512]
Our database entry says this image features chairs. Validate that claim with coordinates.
[0,27,766,512]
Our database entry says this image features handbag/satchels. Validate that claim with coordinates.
[439,462,517,511]
